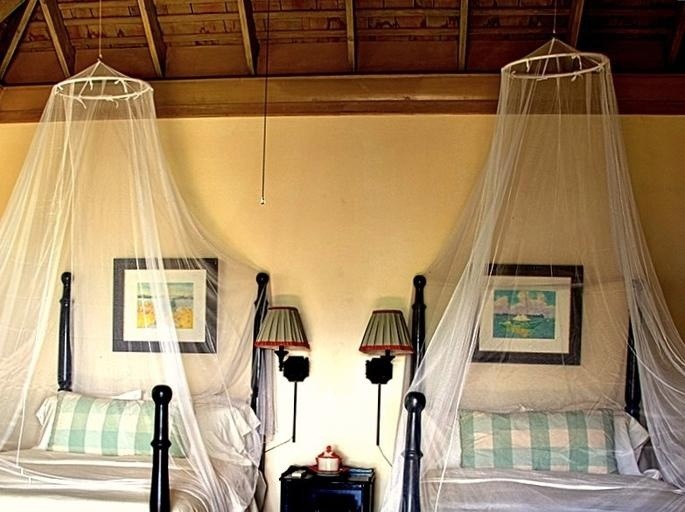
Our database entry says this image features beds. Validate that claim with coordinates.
[410,397,682,511]
[2,391,249,512]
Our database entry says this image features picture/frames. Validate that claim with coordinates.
[471,260,583,367]
[109,255,220,353]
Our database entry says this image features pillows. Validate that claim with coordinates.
[434,406,618,478]
[49,394,186,457]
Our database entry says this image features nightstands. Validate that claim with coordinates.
[282,465,375,510]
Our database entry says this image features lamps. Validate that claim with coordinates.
[359,309,416,386]
[256,305,313,381]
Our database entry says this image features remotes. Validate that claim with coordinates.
[292,469,306,477]
